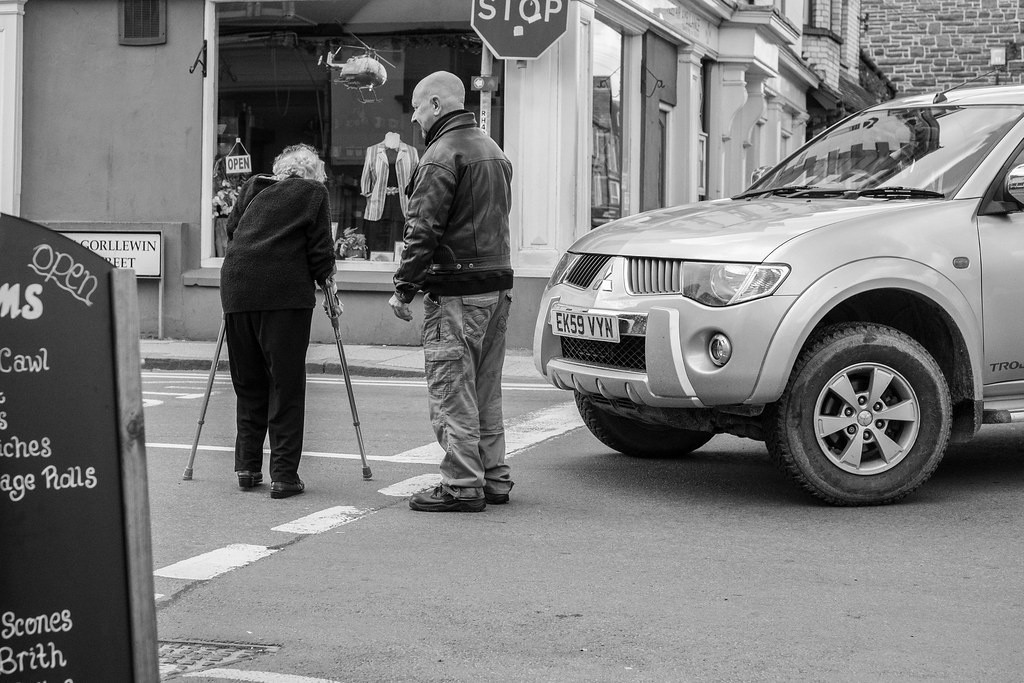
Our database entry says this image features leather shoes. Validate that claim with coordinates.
[408,486,487,514]
[482,487,511,505]
[270,478,306,499]
[236,468,264,488]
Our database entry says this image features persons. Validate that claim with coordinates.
[386,70,515,515]
[359,132,419,259]
[218,143,343,501]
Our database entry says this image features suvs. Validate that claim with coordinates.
[533,70,1024,506]
